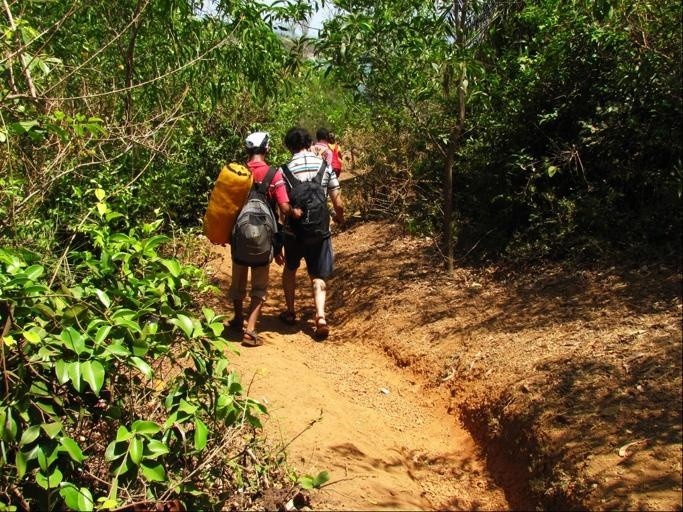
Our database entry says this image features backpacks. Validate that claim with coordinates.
[281,160,330,241]
[331,145,341,178]
[231,163,281,266]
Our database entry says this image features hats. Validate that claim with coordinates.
[245,132,269,152]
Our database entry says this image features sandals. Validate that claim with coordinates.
[316,317,328,337]
[281,311,295,323]
[230,317,242,334]
[242,332,262,346]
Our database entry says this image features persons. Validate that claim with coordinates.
[225,129,302,347]
[326,129,341,177]
[308,127,332,169]
[272,125,345,338]
